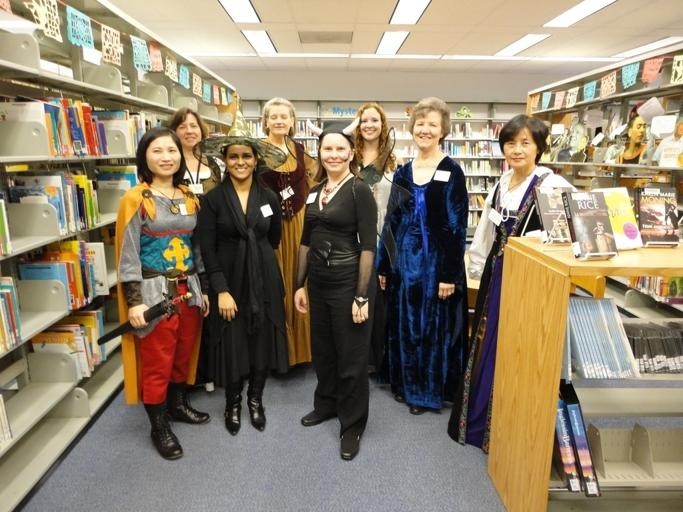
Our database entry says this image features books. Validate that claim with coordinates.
[0,276,22,355]
[97,163,138,191]
[0,97,158,157]
[0,198,13,257]
[561,191,618,261]
[448,122,505,139]
[533,183,576,246]
[468,211,480,228]
[459,159,509,174]
[638,187,679,242]
[553,385,603,499]
[635,185,681,246]
[246,119,264,139]
[468,194,485,209]
[32,310,106,381]
[10,169,101,235]
[466,177,498,191]
[120,72,132,98]
[444,141,492,156]
[562,293,683,381]
[595,186,644,250]
[20,240,96,309]
[0,395,13,444]
[294,119,322,156]
[41,56,73,78]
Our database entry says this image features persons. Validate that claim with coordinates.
[616,103,651,166]
[259,98,323,376]
[192,110,287,436]
[652,121,683,169]
[602,134,625,163]
[556,121,589,163]
[170,107,227,205]
[293,119,377,459]
[551,129,569,161]
[445,114,576,453]
[591,220,614,253]
[572,135,589,163]
[351,103,402,243]
[665,205,679,231]
[114,130,210,459]
[379,97,470,414]
[624,224,637,239]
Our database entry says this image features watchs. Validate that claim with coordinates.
[355,296,368,303]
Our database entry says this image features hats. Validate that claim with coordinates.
[306,118,360,145]
[192,95,287,177]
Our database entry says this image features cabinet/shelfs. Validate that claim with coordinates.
[488,236,683,512]
[490,103,525,188]
[240,100,319,161]
[526,43,683,239]
[319,101,490,242]
[0,0,237,512]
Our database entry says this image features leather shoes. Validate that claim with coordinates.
[340,432,360,461]
[409,406,424,415]
[301,410,336,426]
[394,392,406,402]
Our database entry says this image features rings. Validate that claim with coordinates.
[448,292,451,296]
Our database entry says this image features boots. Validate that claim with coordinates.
[224,377,243,436]
[144,401,182,459]
[247,373,265,432]
[165,383,212,425]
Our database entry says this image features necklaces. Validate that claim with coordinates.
[150,182,181,214]
[321,172,351,208]
